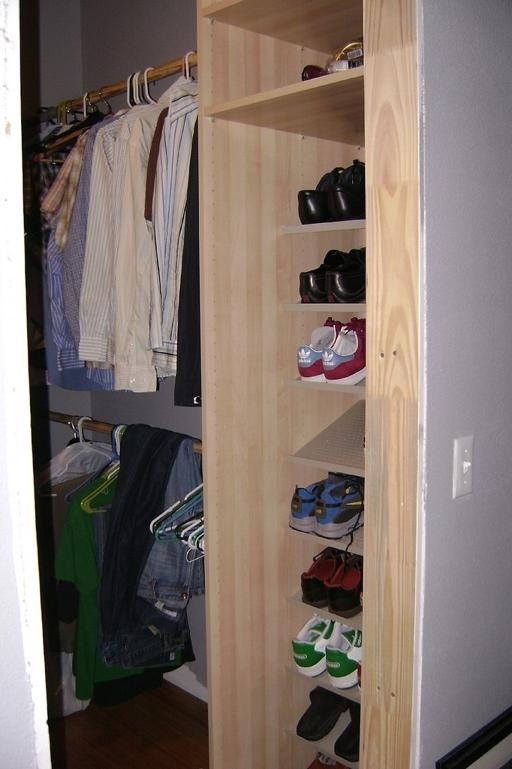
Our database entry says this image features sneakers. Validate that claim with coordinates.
[301,547,363,618]
[291,615,362,689]
[298,317,366,384]
[289,471,364,540]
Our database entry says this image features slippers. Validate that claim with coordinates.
[297,686,360,763]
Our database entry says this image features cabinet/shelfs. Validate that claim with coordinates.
[198,0,417,768]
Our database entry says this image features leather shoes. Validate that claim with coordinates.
[299,247,366,303]
[298,158,365,224]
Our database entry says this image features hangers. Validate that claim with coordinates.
[33,50,198,163]
[38,417,204,566]
